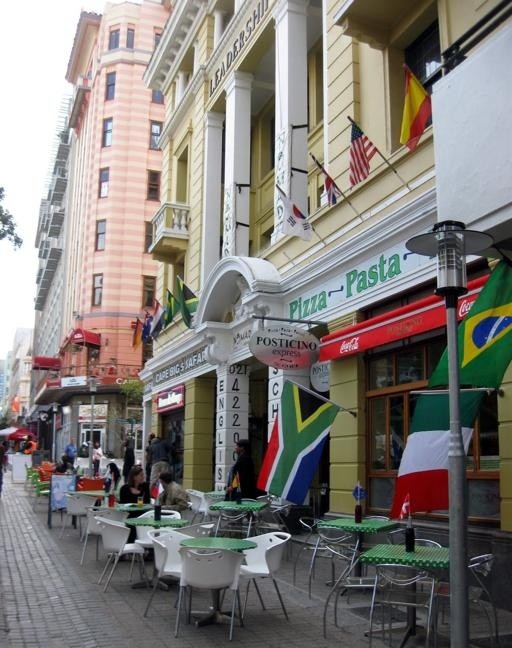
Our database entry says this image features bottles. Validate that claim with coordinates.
[406,516,415,551]
[155,496,162,521]
[105,494,115,508]
[355,495,361,523]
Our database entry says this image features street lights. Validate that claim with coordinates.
[85,375,100,478]
[404,220,495,646]
[50,401,62,464]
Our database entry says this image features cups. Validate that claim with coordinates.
[138,496,143,505]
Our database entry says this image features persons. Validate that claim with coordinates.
[19,433,37,454]
[225,437,254,502]
[52,432,187,544]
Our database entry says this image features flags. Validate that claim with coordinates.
[348,126,379,191]
[391,393,485,518]
[399,70,430,153]
[324,178,343,208]
[428,257,511,388]
[279,191,312,242]
[254,380,341,504]
[129,275,199,350]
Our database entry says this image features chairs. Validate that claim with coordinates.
[21,463,294,643]
[294,516,502,643]
